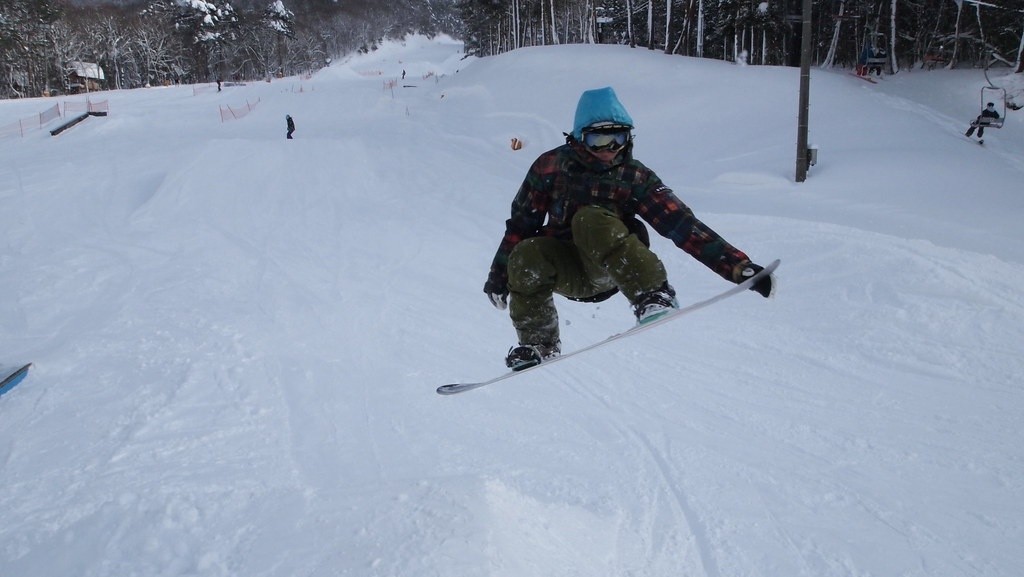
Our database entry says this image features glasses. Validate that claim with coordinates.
[583,131,629,152]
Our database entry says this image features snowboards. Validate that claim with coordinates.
[435,258,783,396]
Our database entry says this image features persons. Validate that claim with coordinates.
[484,87,776,370]
[965,103,999,138]
[216,79,221,91]
[857,44,886,76]
[402,69,405,79]
[286,115,296,139]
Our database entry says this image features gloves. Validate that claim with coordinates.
[487,291,507,310]
[741,263,771,298]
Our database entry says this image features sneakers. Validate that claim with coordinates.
[633,284,679,323]
[506,340,562,368]
